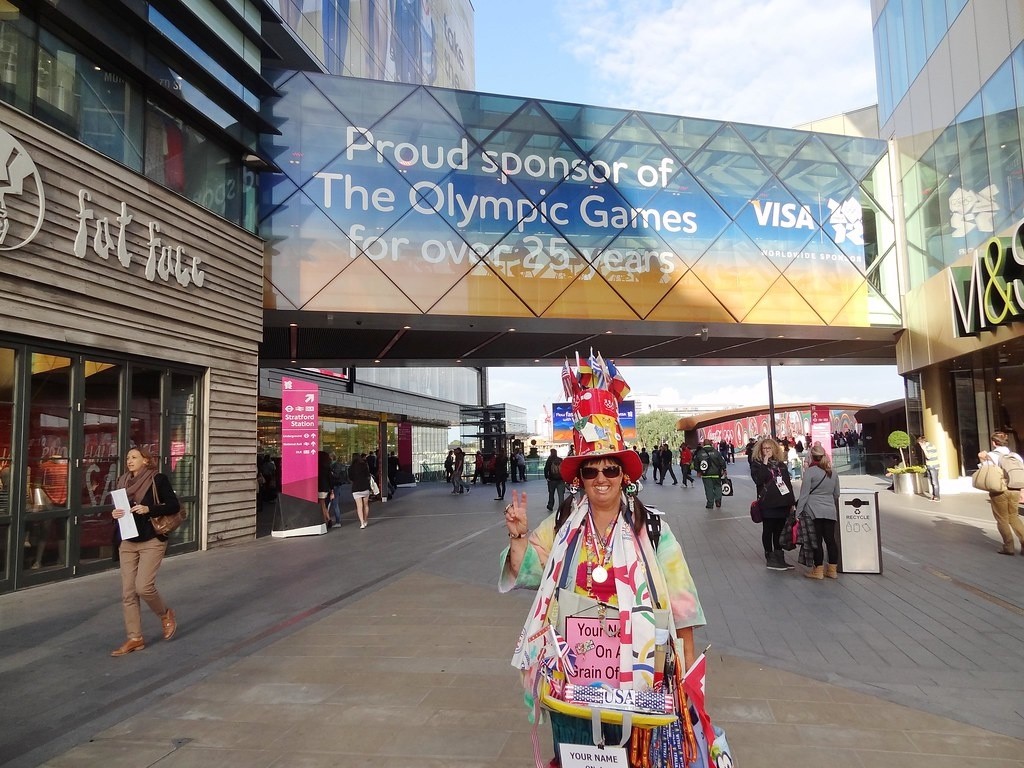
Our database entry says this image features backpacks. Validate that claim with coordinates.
[990,450,1024,488]
[550,457,562,480]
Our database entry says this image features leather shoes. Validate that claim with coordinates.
[161,608,177,641]
[110,638,145,656]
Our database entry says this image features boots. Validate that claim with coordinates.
[823,562,837,579]
[765,550,795,570]
[803,563,824,579]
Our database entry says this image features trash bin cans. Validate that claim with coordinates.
[836,488,883,574]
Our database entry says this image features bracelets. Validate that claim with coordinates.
[508,530,530,539]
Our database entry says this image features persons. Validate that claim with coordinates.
[977,432,1024,556]
[111,446,180,657]
[256,454,282,512]
[632,439,735,508]
[320,450,399,529]
[917,436,940,502]
[445,445,574,511]
[746,428,863,578]
[24,434,67,569]
[498,415,707,717]
[1000,404,1011,431]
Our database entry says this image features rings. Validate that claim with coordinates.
[503,504,513,514]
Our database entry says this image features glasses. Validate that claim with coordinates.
[582,466,622,479]
[762,447,772,451]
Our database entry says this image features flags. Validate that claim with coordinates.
[680,651,714,745]
[540,628,574,693]
[562,355,631,404]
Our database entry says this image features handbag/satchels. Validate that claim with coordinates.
[750,498,763,523]
[721,476,733,496]
[370,475,380,495]
[688,719,734,768]
[795,499,798,510]
[148,470,186,536]
[972,454,1007,492]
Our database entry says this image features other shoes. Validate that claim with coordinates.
[547,505,553,511]
[706,506,713,509]
[716,502,721,507]
[928,496,941,503]
[360,522,368,528]
[1020,542,1024,555]
[656,482,662,485]
[691,480,695,487]
[328,520,342,527]
[997,549,1015,555]
[495,497,504,501]
[672,482,678,485]
[681,484,687,487]
[451,487,471,495]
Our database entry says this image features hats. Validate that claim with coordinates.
[559,388,643,482]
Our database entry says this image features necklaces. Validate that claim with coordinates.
[589,506,615,551]
